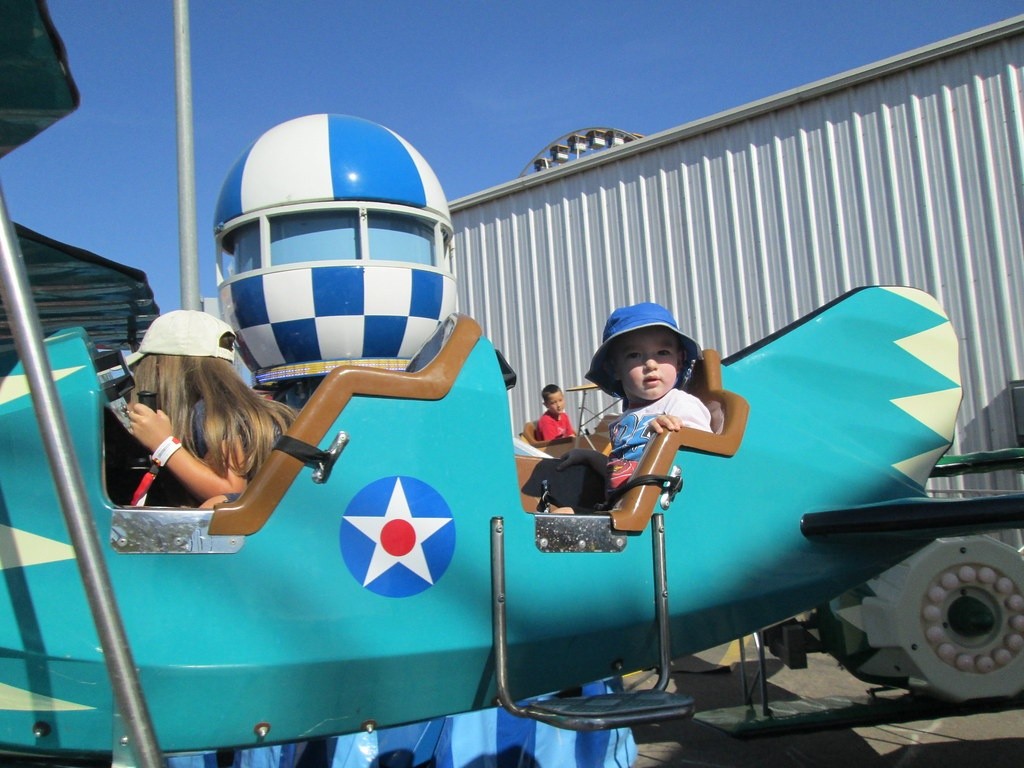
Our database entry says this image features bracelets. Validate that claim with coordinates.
[152,436,181,466]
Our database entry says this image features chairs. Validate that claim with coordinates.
[521,421,577,448]
[514,350,749,533]
[539,138,623,170]
[211,311,479,531]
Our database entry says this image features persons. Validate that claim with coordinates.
[550,303,714,513]
[537,384,576,441]
[125,310,298,509]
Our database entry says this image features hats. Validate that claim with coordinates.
[584,302,704,398]
[124,310,237,371]
